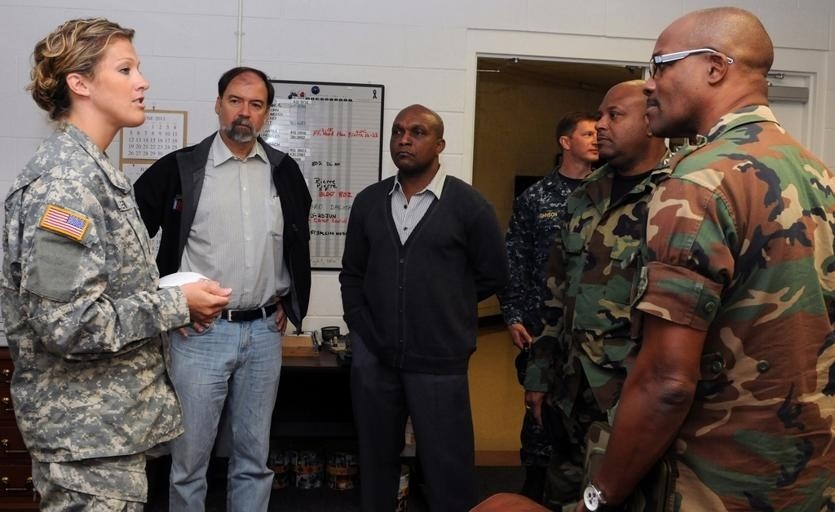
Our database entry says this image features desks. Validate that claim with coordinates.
[284,346,350,375]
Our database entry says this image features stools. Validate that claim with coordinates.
[469,490,551,512]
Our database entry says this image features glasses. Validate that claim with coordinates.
[649,48,733,79]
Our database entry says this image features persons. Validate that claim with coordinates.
[496,112,602,503]
[576,8,835,512]
[336,104,506,510]
[132,66,312,512]
[515,80,675,510]
[1,16,231,511]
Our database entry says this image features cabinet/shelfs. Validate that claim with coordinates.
[0,344,39,512]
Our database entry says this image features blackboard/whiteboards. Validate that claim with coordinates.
[248,79,385,270]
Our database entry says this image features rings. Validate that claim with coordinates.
[526,404,532,409]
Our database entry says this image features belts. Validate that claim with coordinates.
[219,304,277,322]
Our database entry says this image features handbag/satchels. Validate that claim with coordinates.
[580,421,676,512]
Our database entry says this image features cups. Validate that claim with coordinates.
[321,326,340,343]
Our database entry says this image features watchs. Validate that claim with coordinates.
[584,479,607,512]
